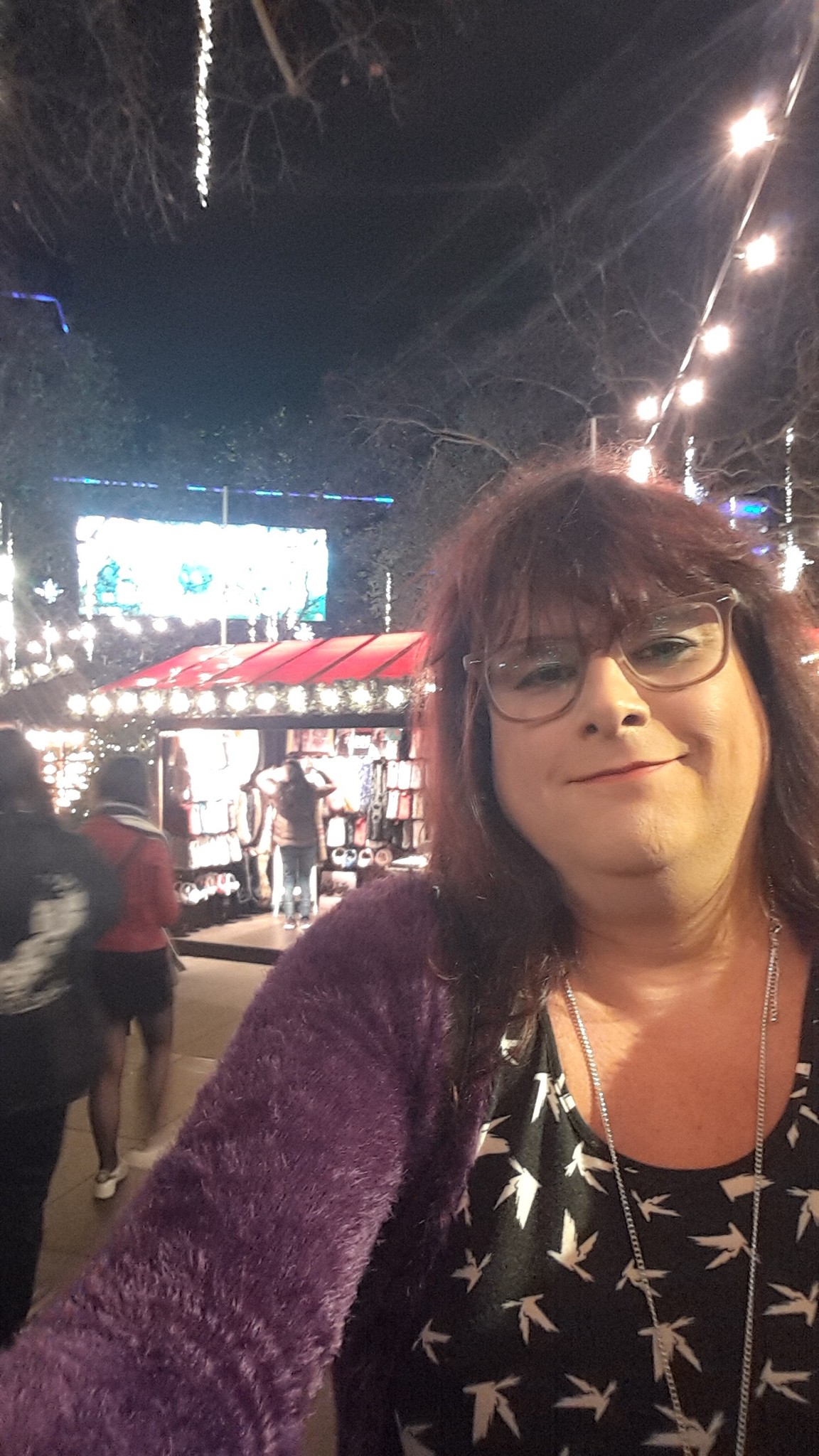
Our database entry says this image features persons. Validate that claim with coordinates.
[0,726,338,1353]
[0,467,819,1455]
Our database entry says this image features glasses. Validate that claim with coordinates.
[462,586,741,724]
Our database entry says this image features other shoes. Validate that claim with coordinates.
[299,916,308,928]
[284,918,297,930]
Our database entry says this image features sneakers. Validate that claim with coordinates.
[123,1144,161,1170]
[95,1158,128,1198]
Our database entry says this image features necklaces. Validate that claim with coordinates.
[559,898,784,1455]
